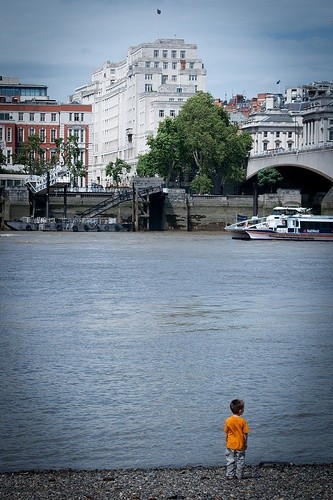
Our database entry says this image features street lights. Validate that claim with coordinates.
[43,167,51,218]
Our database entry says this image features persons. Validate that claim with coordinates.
[224,400,248,479]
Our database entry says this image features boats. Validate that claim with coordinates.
[224,205,314,242]
[244,213,333,242]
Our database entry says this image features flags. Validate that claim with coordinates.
[156,9,161,15]
[179,59,185,70]
